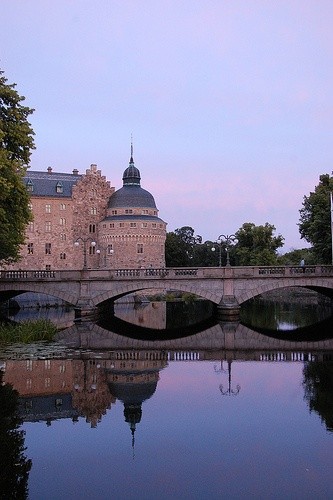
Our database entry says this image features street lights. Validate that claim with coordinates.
[214,360,228,373]
[212,243,229,267]
[74,237,97,270]
[97,360,115,372]
[97,246,114,268]
[219,361,242,396]
[74,359,98,393]
[217,234,239,267]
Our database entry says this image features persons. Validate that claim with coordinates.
[300,257,306,274]
